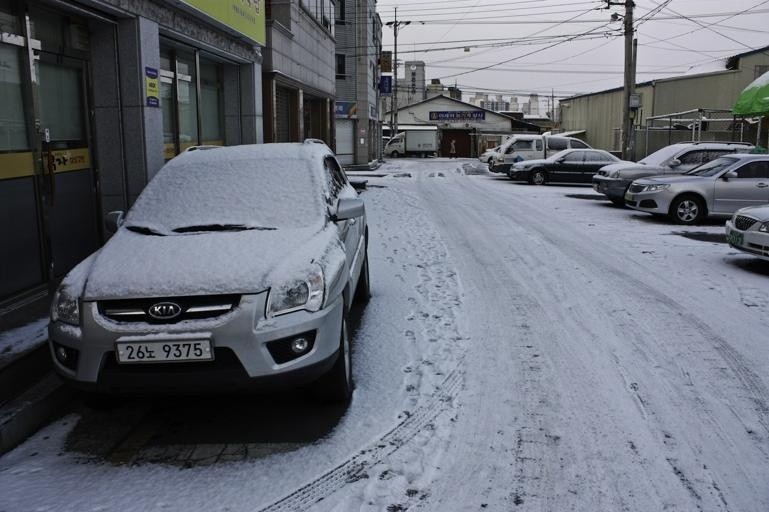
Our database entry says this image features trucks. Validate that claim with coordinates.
[384,129,440,158]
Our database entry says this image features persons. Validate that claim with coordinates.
[450,138,457,159]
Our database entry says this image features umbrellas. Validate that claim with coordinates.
[731,68,769,115]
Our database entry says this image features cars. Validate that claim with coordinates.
[48,138,368,406]
[480,134,769,261]
